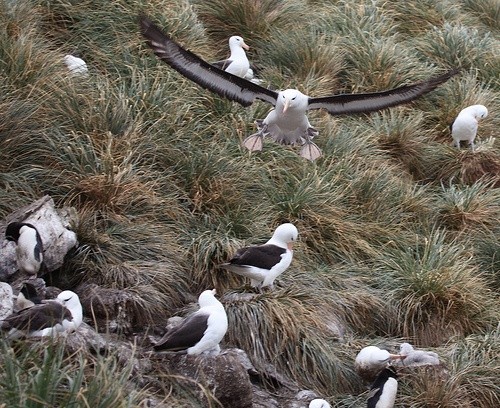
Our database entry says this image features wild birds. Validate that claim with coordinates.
[216,223,299,295]
[0,282,82,338]
[60,55,89,78]
[355,345,404,378]
[5,221,50,280]
[308,369,399,408]
[209,36,250,79]
[449,104,489,154]
[154,288,229,357]
[135,6,458,163]
[394,342,444,367]
